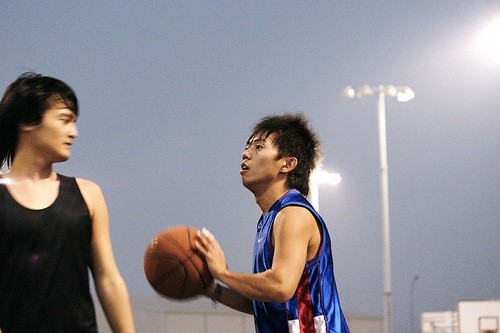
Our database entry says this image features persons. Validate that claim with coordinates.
[195,113,351,333]
[0,71,137,333]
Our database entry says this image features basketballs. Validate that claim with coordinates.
[144,226,214,302]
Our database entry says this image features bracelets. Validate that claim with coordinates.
[212,283,223,302]
